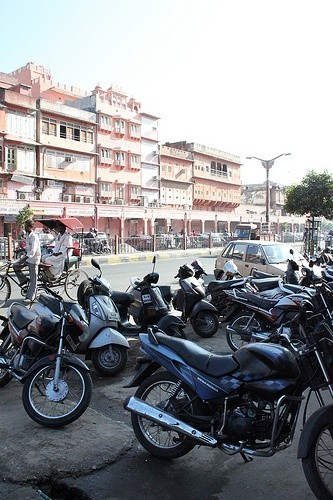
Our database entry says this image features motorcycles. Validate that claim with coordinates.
[0,302,94,429]
[124,299,333,500]
[192,255,333,354]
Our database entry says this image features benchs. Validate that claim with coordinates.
[47,242,82,276]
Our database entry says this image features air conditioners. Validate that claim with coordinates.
[18,193,28,200]
[62,195,94,203]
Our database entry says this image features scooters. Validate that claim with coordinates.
[77,256,187,342]
[127,263,219,337]
[28,259,130,377]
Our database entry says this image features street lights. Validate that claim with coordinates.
[245,152,291,221]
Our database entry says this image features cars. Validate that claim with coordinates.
[214,240,323,282]
[1,225,333,258]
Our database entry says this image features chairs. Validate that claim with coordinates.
[266,247,274,258]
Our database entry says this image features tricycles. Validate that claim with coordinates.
[0,218,89,308]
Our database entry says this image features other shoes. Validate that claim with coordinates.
[18,278,28,286]
[22,298,30,303]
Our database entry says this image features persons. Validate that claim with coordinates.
[11,217,41,303]
[301,227,333,248]
[41,223,73,283]
[165,228,260,244]
[14,226,102,253]
